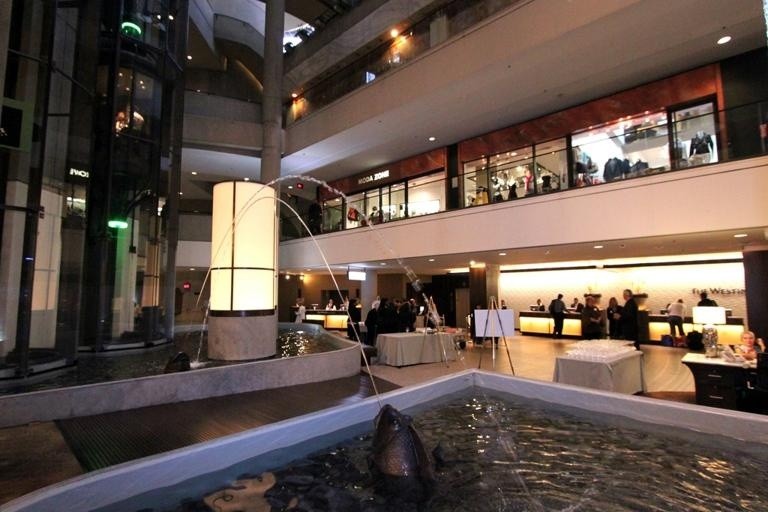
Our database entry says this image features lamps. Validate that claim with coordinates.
[208,177,278,316]
[691,305,727,356]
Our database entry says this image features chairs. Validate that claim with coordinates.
[744,352,767,411]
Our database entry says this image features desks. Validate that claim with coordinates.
[554,348,646,397]
[375,331,459,367]
[567,337,636,350]
[680,350,767,413]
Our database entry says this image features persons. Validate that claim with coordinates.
[666,299,687,336]
[501,300,507,310]
[690,131,713,164]
[571,290,641,349]
[346,298,361,340]
[697,292,718,306]
[326,300,336,310]
[360,206,380,227]
[492,166,552,202]
[686,331,765,361]
[296,303,305,322]
[548,294,570,337]
[364,296,418,348]
[536,298,545,311]
